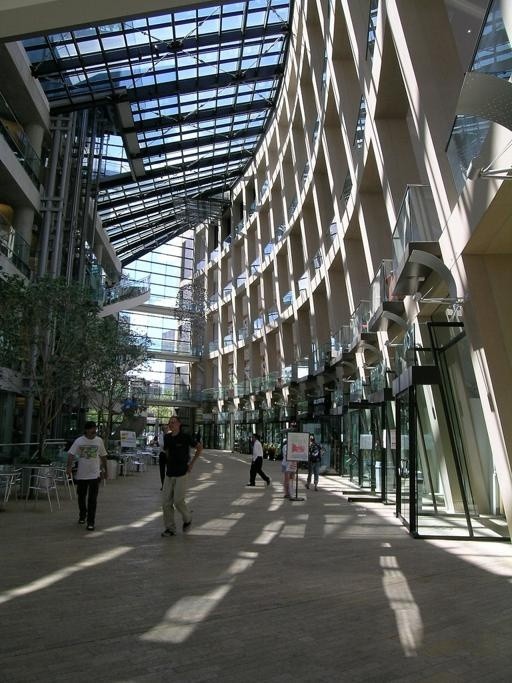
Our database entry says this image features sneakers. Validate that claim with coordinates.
[162,529,176,537]
[246,477,271,487]
[182,522,191,532]
[78,518,95,530]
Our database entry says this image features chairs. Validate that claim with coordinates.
[0,460,76,513]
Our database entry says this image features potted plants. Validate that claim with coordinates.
[104,454,120,479]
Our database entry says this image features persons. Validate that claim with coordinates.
[65,421,109,531]
[104,281,113,305]
[303,435,323,492]
[150,436,160,447]
[246,434,271,486]
[158,424,171,491]
[162,416,203,537]
[280,438,298,498]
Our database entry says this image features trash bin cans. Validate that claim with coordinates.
[375,461,394,492]
[106,456,117,479]
